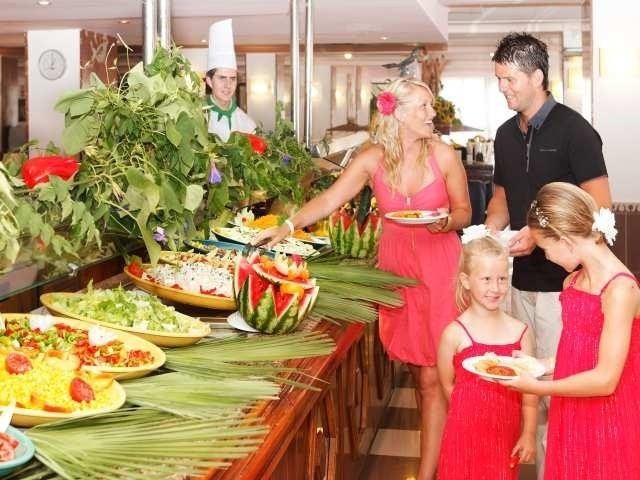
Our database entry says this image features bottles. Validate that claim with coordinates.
[467,134,495,162]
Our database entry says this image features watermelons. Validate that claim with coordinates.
[233,253,320,337]
[328,210,382,260]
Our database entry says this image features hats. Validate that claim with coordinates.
[207,19,238,73]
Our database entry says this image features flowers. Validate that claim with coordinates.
[376,92,397,116]
[592,207,618,247]
[433,96,455,124]
[461,224,490,244]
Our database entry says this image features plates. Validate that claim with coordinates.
[223,309,262,333]
[461,352,547,383]
[180,235,281,260]
[211,224,319,258]
[0,311,166,380]
[0,350,130,475]
[39,289,209,347]
[227,215,331,246]
[157,250,220,266]
[120,263,241,310]
[462,230,523,251]
[382,210,452,228]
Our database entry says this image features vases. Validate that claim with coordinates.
[435,122,450,135]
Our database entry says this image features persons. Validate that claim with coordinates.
[434,236,538,480]
[480,33,612,480]
[246,74,474,479]
[493,181,640,480]
[199,17,257,144]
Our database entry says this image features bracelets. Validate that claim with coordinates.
[285,219,295,235]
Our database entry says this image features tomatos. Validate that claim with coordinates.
[69,376,96,404]
[4,351,34,377]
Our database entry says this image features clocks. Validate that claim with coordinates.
[39,50,66,81]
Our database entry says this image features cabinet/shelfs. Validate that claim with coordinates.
[30,260,404,480]
